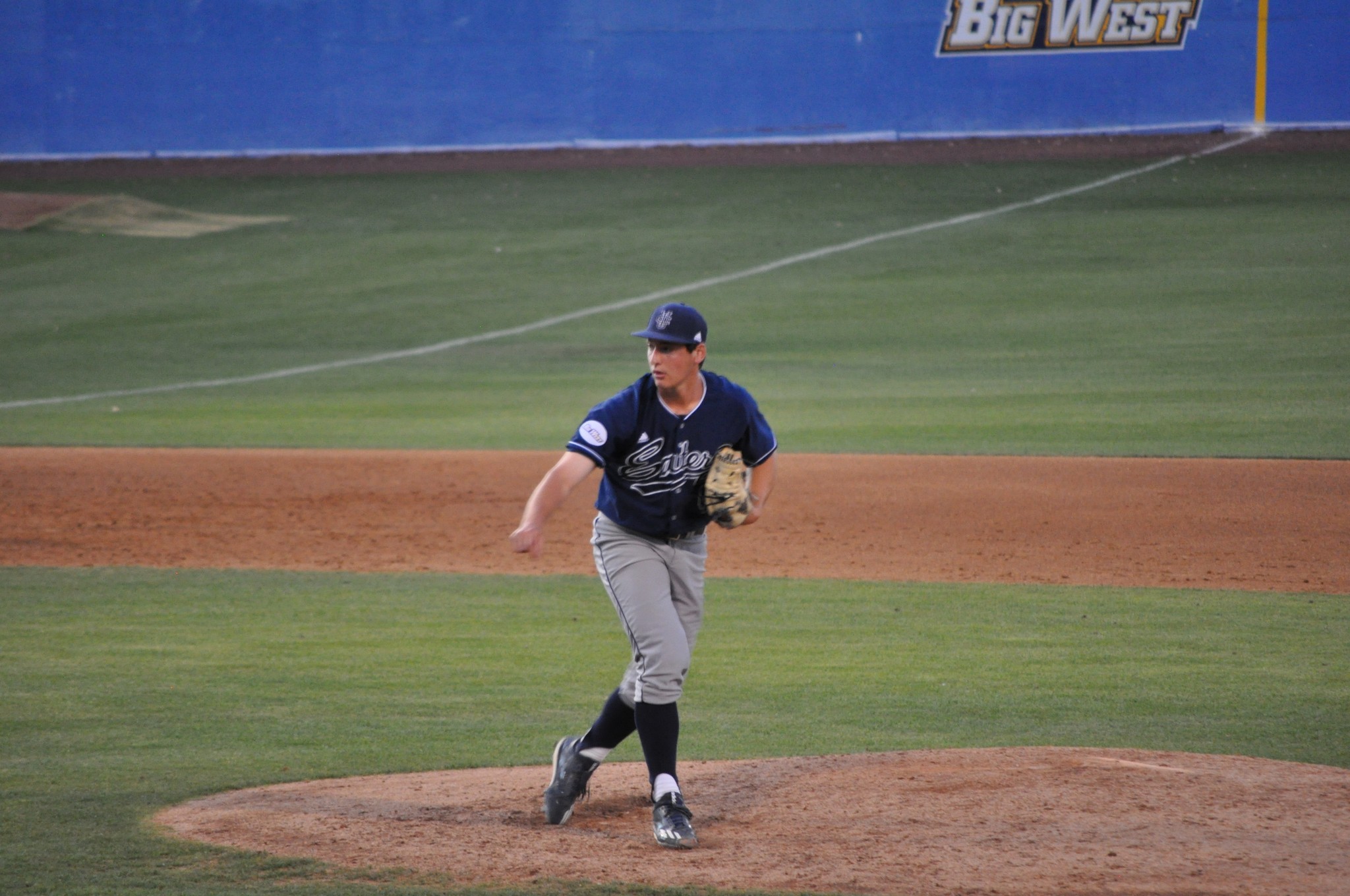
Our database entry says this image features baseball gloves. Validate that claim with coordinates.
[704,447,754,530]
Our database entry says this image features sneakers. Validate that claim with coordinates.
[652,791,699,850]
[543,734,594,827]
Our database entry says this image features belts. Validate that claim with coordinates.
[662,524,706,540]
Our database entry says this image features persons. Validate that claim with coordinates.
[509,303,781,849]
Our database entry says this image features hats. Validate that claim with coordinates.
[630,301,708,345]
